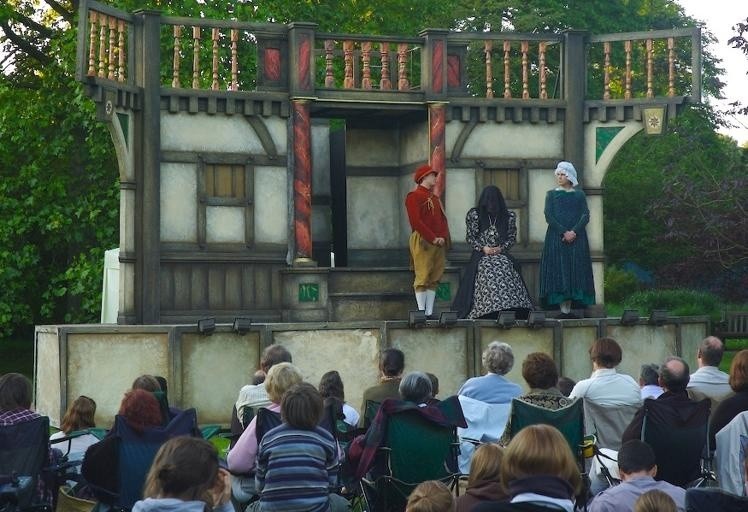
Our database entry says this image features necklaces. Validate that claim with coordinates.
[487,212,497,236]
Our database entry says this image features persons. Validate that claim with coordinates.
[406,480,455,511]
[405,164,449,319]
[49,395,109,488]
[540,162,596,318]
[0,372,53,506]
[621,335,746,489]
[634,488,676,511]
[455,186,534,315]
[456,443,509,511]
[83,375,182,503]
[340,336,641,498]
[227,343,360,511]
[471,424,582,511]
[588,440,686,511]
[132,435,235,511]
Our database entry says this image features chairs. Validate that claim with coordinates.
[594,394,718,495]
[1,397,223,512]
[345,394,468,512]
[211,393,344,510]
[460,394,590,511]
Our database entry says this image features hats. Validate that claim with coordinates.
[416,166,438,184]
[555,162,578,186]
